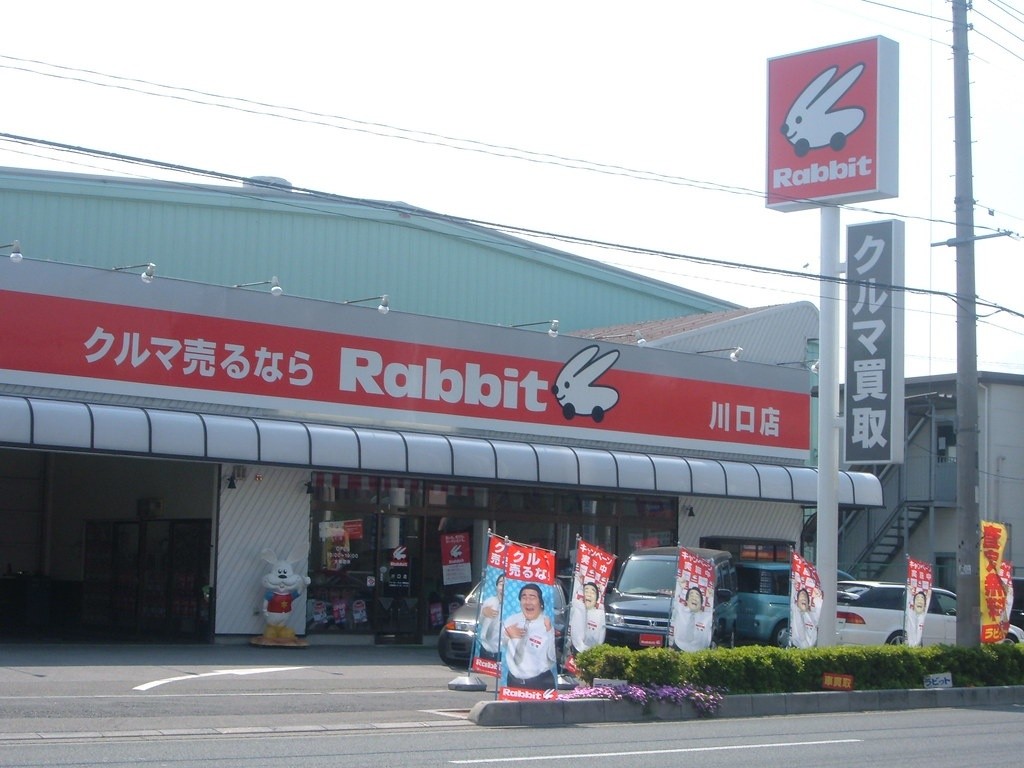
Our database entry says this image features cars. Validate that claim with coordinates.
[438,572,571,668]
[835,580,1024,647]
[307,566,465,636]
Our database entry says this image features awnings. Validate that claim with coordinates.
[0,391,885,534]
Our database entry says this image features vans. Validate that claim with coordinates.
[601,543,740,652]
[731,556,861,648]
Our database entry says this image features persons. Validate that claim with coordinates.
[570,582,606,660]
[671,587,712,652]
[906,589,931,646]
[501,583,556,691]
[790,588,823,649]
[478,574,502,662]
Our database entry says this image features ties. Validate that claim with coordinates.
[582,610,589,643]
[513,619,531,666]
[486,601,502,640]
[913,614,919,642]
[686,612,696,643]
[798,611,806,640]
